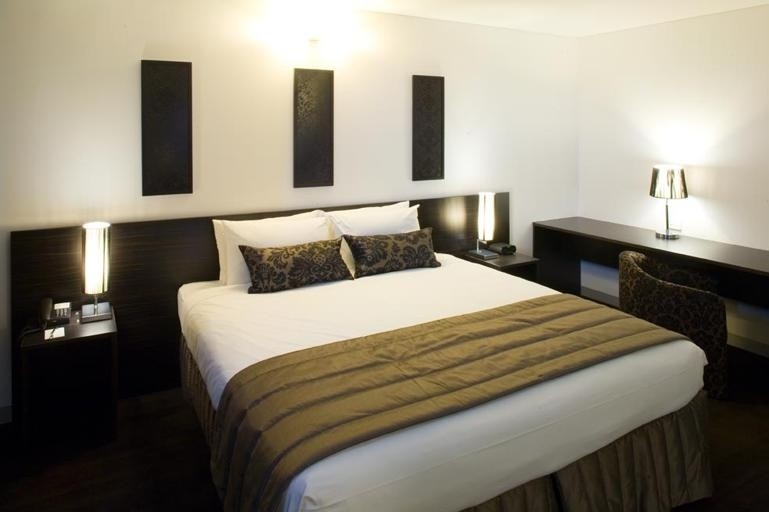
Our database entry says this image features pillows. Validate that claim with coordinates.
[328,201,410,225]
[346,227,441,279]
[212,209,324,286]
[238,238,354,295]
[223,217,329,286]
[334,203,422,236]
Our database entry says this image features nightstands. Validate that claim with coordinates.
[465,192,499,260]
[463,252,541,285]
[22,307,119,462]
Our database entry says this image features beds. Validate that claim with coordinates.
[176,250,714,510]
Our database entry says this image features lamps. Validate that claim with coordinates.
[79,221,112,323]
[650,166,688,240]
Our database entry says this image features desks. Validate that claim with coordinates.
[532,216,768,310]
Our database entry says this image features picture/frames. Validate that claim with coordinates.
[412,75,444,182]
[294,68,335,188]
[140,59,193,196]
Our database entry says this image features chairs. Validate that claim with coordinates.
[618,251,728,403]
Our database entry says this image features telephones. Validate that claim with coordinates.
[37,297,72,328]
